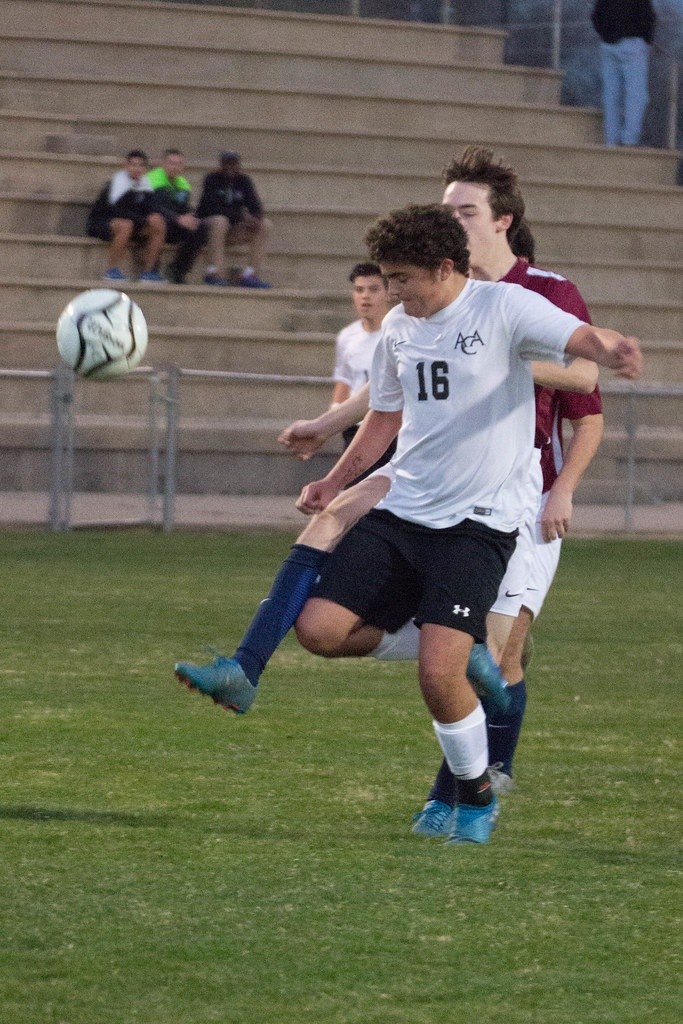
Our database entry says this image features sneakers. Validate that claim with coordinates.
[444,795,500,845]
[468,644,512,715]
[173,657,259,715]
[487,761,518,797]
[409,800,452,838]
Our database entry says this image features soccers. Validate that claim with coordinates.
[56,288,150,381]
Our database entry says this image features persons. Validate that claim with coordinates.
[588,1,659,154]
[174,148,644,848]
[88,146,196,286]
[198,146,271,291]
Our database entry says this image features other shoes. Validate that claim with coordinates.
[233,272,270,288]
[103,269,128,282]
[204,274,227,286]
[165,268,187,285]
[138,270,170,284]
